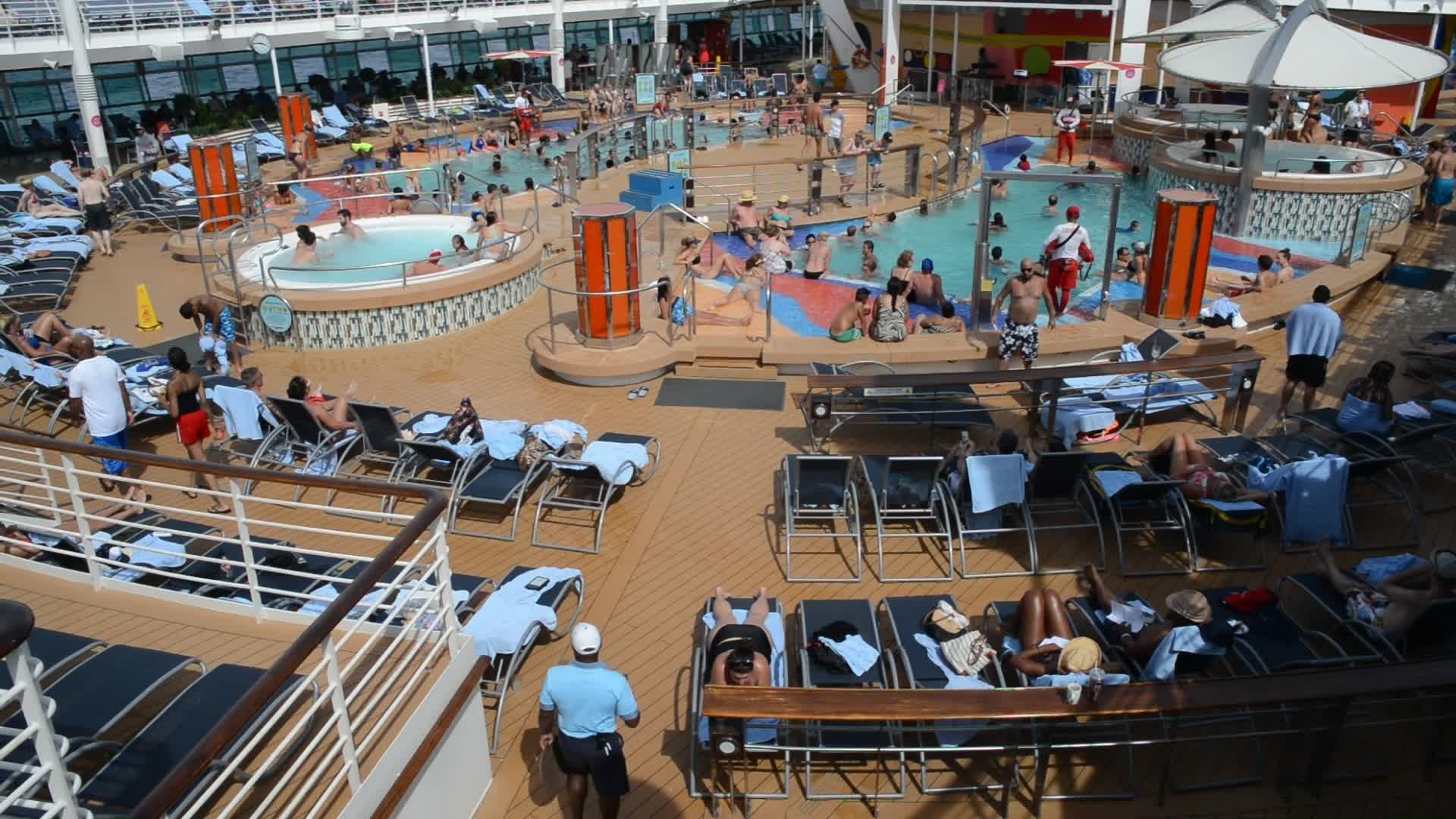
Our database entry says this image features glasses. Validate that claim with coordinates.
[306,380,310,388]
[806,240,815,244]
[1023,268,1033,272]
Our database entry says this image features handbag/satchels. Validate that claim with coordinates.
[939,629,995,675]
[921,608,967,642]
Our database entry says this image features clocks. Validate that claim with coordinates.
[250,33,271,54]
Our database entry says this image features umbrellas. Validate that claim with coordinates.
[479,48,560,87]
[1050,58,1151,153]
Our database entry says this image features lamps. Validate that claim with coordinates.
[449,5,458,22]
[43,58,59,72]
[640,11,649,19]
[207,19,223,42]
[525,20,534,28]
[385,26,413,42]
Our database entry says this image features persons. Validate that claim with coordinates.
[997,588,1102,679]
[0,36,1455,514]
[1312,534,1456,643]
[706,586,775,687]
[1082,561,1211,665]
[540,622,640,818]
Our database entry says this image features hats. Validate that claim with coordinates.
[1057,636,1101,673]
[1066,206,1080,219]
[1065,96,1075,102]
[777,195,790,202]
[429,251,441,264]
[830,100,839,106]
[1132,241,1145,251]
[570,623,601,655]
[921,258,934,271]
[687,238,701,245]
[1168,589,1211,624]
[1429,550,1456,579]
[740,190,757,202]
[884,132,892,143]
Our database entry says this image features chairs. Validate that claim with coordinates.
[0,82,565,166]
[0,627,319,819]
[670,66,806,101]
[0,160,659,756]
[737,32,810,57]
[692,328,1456,818]
[1321,122,1456,166]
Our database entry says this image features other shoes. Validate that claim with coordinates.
[131,493,152,502]
[803,182,884,213]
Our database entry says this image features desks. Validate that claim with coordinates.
[83,138,132,166]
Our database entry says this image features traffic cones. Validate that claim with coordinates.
[714,55,721,76]
[134,283,163,333]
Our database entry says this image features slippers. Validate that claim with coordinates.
[99,477,115,491]
[638,386,648,397]
[628,388,637,400]
[182,490,198,499]
[208,504,232,513]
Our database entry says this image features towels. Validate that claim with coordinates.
[442,83,517,116]
[27,526,188,583]
[150,131,286,195]
[310,104,377,140]
[186,0,215,16]
[237,1,256,13]
[0,159,95,295]
[1197,295,1248,329]
[1284,302,1341,360]
[0,328,358,477]
[946,452,1029,542]
[1093,469,1144,501]
[413,413,651,486]
[1036,341,1221,451]
[996,583,1281,689]
[1333,393,1456,435]
[818,633,881,678]
[695,607,787,748]
[1355,551,1430,590]
[1393,139,1428,160]
[690,71,786,101]
[295,564,583,668]
[216,595,264,607]
[912,631,997,751]
[1195,448,1354,549]
[1319,112,1339,128]
